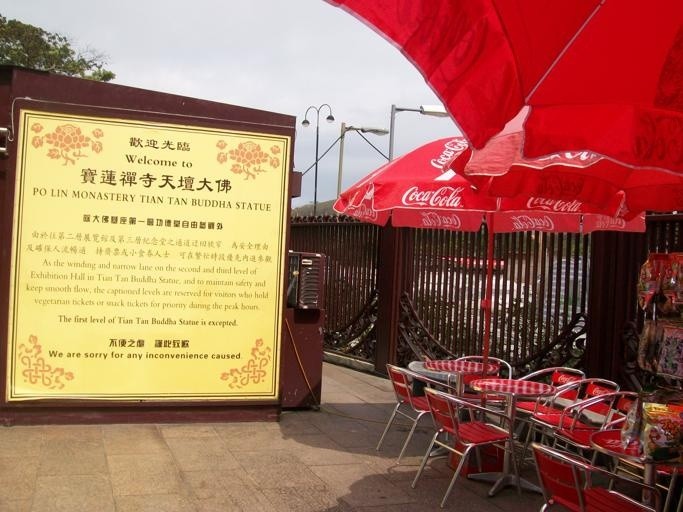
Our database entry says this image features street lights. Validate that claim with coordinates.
[336,122,389,204]
[301,104,333,215]
[388,104,450,163]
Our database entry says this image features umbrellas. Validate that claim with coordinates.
[335,134,647,378]
[440,104,683,220]
[324,2,683,177]
